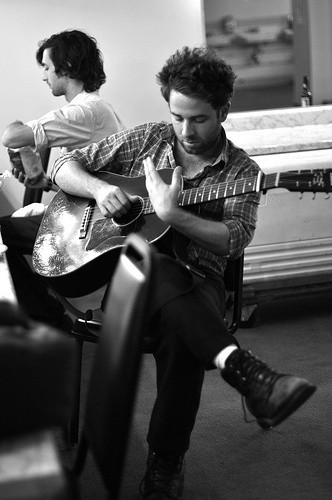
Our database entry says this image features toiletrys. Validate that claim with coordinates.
[299,74,313,108]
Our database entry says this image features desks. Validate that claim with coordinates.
[0,189,67,499]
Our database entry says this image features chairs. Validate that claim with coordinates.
[57,230,154,499]
[69,249,244,470]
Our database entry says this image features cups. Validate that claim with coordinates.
[7,145,43,180]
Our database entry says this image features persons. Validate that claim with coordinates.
[2,31,134,326]
[51,44,317,499]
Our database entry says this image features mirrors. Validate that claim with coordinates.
[187,0,332,133]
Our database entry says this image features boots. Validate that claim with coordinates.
[220,347,318,432]
[143,443,185,500]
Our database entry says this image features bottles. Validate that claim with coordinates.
[300,75,312,108]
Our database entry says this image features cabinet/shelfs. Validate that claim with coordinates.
[205,13,301,89]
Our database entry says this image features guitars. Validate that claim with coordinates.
[32,165,332,300]
